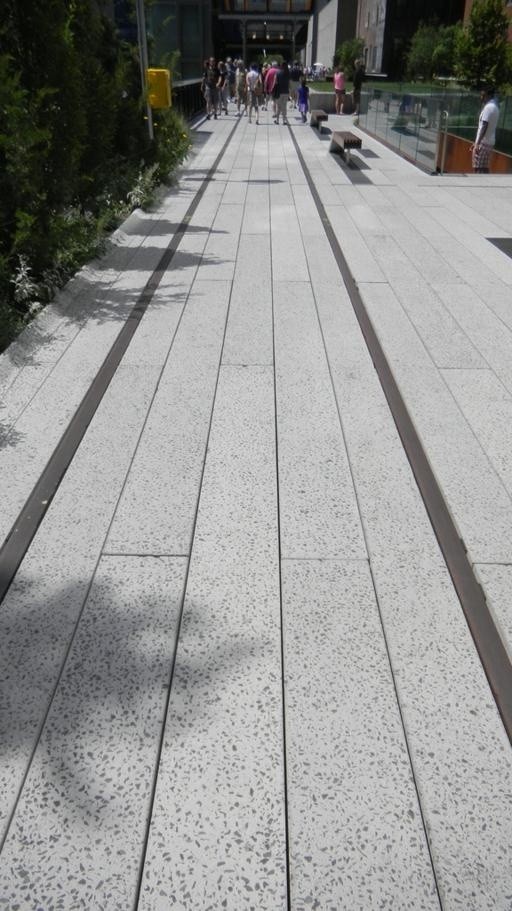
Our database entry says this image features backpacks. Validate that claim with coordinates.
[252,73,264,97]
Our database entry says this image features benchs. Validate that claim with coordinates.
[329,130,362,164]
[310,109,328,134]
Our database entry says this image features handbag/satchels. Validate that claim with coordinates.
[271,72,281,99]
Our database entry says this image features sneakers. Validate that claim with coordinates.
[206,108,307,125]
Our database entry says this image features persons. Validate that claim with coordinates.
[332,63,346,115]
[350,59,364,116]
[198,55,327,125]
[468,84,501,174]
